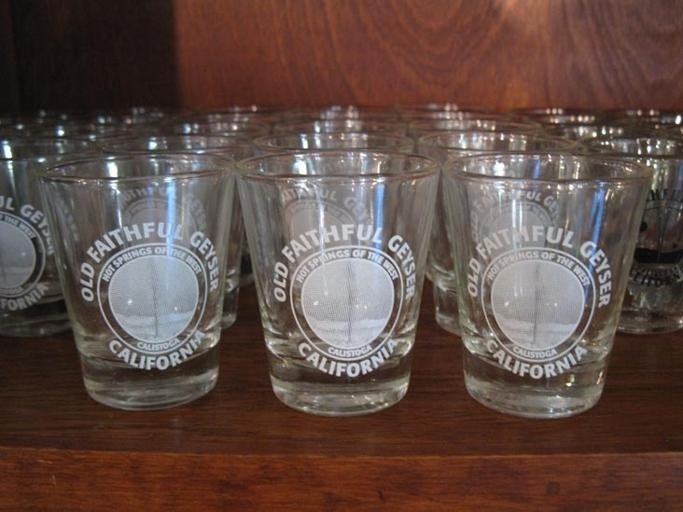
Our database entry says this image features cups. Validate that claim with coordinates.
[34,156,235,414]
[442,158,655,420]
[0,101,683,339]
[235,151,441,420]
